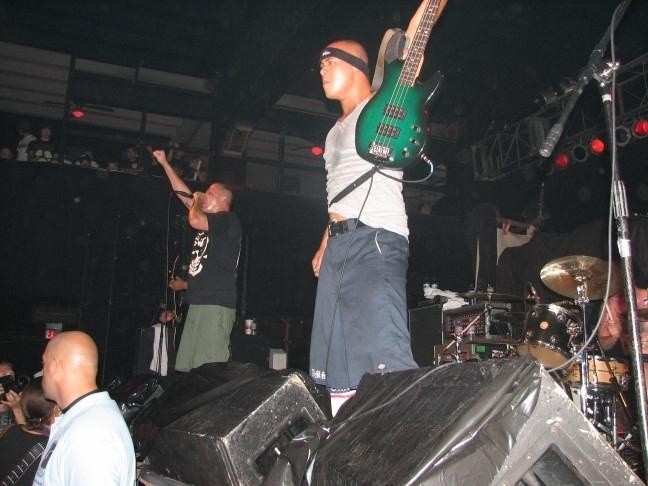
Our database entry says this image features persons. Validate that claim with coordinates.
[130,199,184,387]
[1,114,210,186]
[596,253,647,353]
[0,373,66,486]
[305,35,424,421]
[0,353,29,438]
[151,146,244,375]
[29,329,138,486]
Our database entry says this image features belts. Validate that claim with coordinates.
[327,217,366,238]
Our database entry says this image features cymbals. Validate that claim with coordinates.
[452,291,520,301]
[540,255,620,300]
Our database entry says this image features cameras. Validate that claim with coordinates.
[0,375,31,403]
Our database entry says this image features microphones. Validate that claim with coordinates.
[531,286,541,304]
[178,191,195,198]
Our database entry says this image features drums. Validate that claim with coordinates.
[515,303,585,368]
[561,355,630,393]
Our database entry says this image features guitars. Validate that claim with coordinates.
[355,0,447,170]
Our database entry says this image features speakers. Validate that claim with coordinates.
[108,375,165,426]
[303,359,646,486]
[133,367,327,486]
